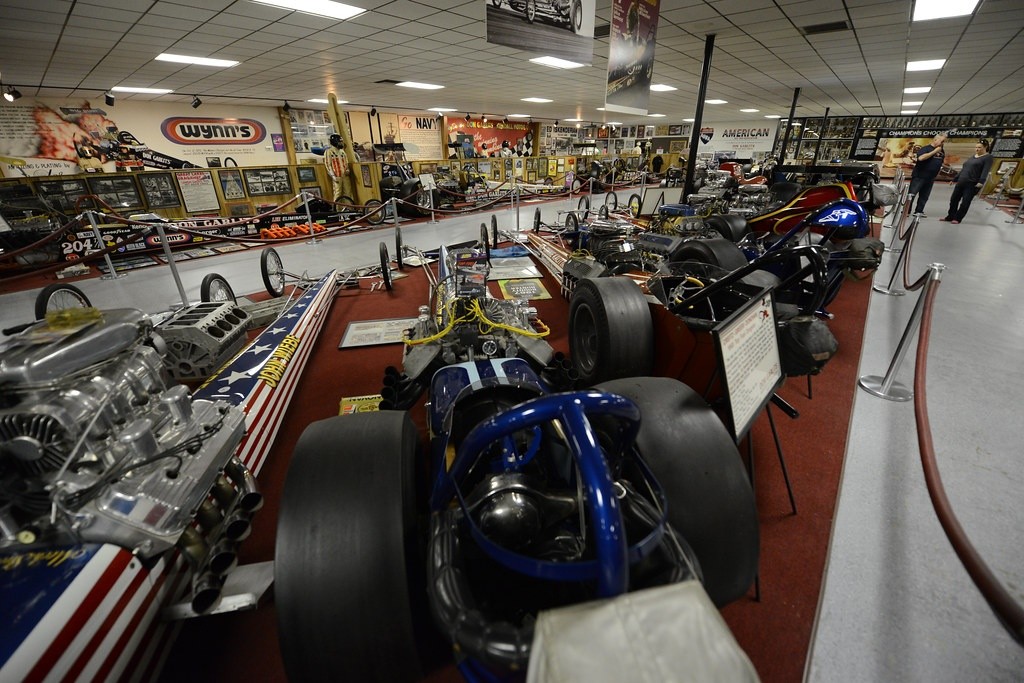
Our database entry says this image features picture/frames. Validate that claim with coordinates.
[626,157,640,167]
[337,317,421,350]
[598,125,691,153]
[361,165,372,187]
[289,109,353,154]
[0,167,323,219]
[996,160,1020,175]
[97,242,267,273]
[418,157,587,202]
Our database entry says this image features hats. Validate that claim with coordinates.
[637,142,641,146]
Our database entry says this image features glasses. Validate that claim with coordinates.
[977,140,984,144]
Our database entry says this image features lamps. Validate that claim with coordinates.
[501,118,508,125]
[190,94,201,108]
[105,89,115,106]
[527,118,532,125]
[371,105,376,116]
[3,85,23,102]
[283,99,290,111]
[482,115,487,123]
[464,113,470,122]
[437,111,443,120]
[554,120,558,128]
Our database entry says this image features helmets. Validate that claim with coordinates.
[330,133,344,149]
[502,141,508,147]
[482,143,488,149]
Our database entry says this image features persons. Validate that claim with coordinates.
[480,143,489,158]
[939,138,993,225]
[602,146,607,154]
[904,134,945,217]
[324,133,354,211]
[500,141,511,158]
[632,142,641,154]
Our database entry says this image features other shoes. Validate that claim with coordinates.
[913,211,927,218]
[939,218,945,221]
[339,215,344,221]
[952,220,958,224]
[345,215,350,221]
[901,210,910,217]
